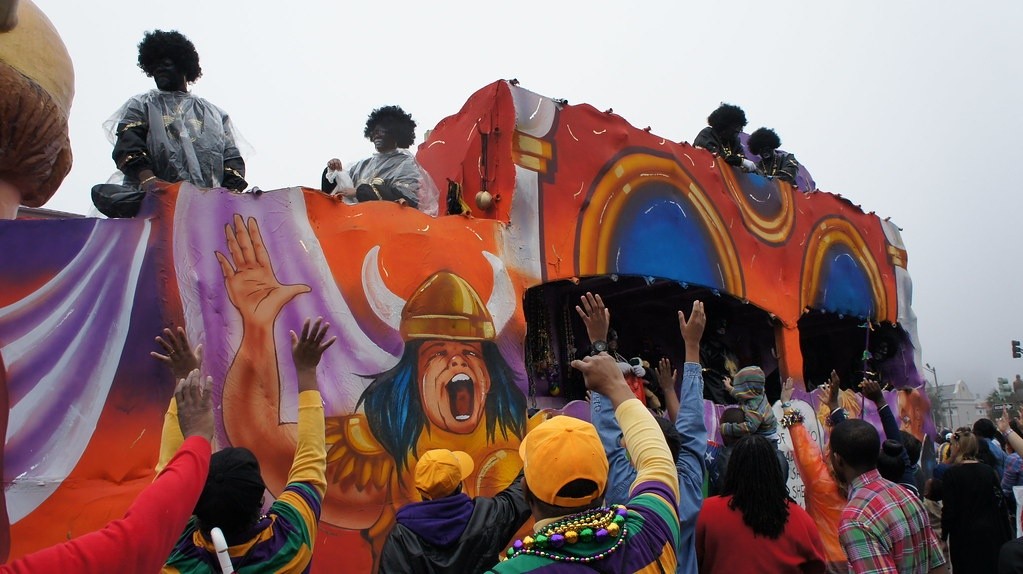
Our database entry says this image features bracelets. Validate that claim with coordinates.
[830,407,841,416]
[1004,429,1013,437]
[141,176,156,185]
[780,409,803,428]
[878,404,888,412]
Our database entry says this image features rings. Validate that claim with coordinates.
[167,350,175,356]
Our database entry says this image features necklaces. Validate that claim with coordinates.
[762,155,776,176]
[502,504,628,562]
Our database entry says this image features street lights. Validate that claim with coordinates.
[924,363,940,397]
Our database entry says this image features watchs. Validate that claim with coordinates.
[781,401,791,407]
[590,340,608,353]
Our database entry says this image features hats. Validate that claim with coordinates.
[519,414,610,507]
[414,449,475,498]
[198,448,264,542]
[973,418,994,439]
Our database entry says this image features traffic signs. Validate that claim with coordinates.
[992,404,1014,411]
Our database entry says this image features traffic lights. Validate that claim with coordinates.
[1012,340,1022,358]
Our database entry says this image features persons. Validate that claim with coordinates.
[692,105,756,172]
[103,30,246,190]
[0,369,215,574]
[656,358,1023,574]
[322,105,440,215]
[151,316,337,574]
[747,127,798,186]
[576,292,708,574]
[487,352,681,574]
[377,413,552,574]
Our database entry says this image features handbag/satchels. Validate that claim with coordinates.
[1012,485,1023,537]
